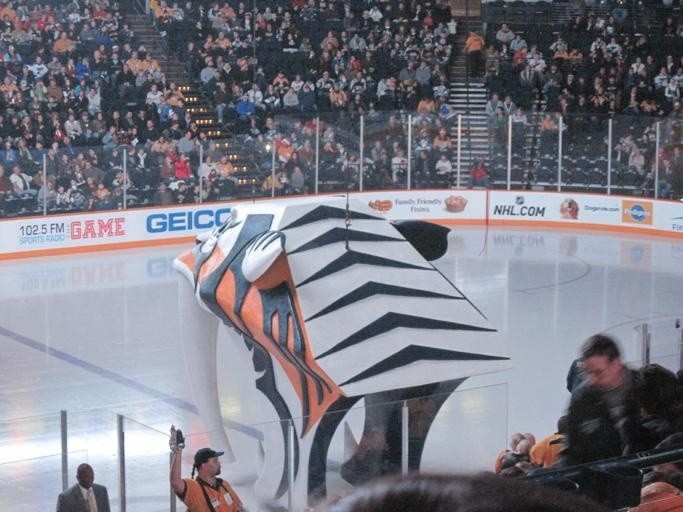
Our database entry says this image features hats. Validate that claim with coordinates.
[194,448,224,465]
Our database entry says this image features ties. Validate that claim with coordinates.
[86,489,96,512]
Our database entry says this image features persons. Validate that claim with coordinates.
[302,334,683,512]
[57,463,111,512]
[170,424,244,512]
[0,0,683,210]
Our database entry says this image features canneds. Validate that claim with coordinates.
[177,430,185,450]
